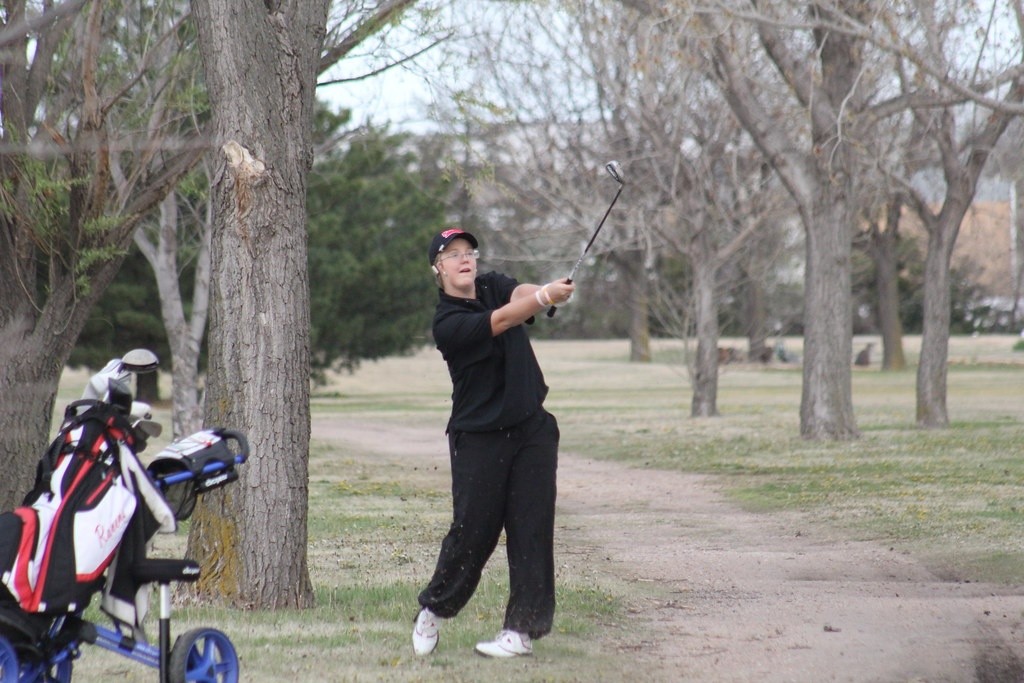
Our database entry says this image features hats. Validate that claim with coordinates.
[429,228,479,264]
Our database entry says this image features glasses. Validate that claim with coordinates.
[436,249,481,262]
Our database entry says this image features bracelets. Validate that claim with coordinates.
[544,284,554,305]
[535,291,546,307]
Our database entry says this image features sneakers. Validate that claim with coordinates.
[474,630,534,659]
[413,608,440,658]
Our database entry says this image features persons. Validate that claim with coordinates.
[412,228,576,660]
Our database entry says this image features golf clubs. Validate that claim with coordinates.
[77,347,165,440]
[546,160,626,318]
